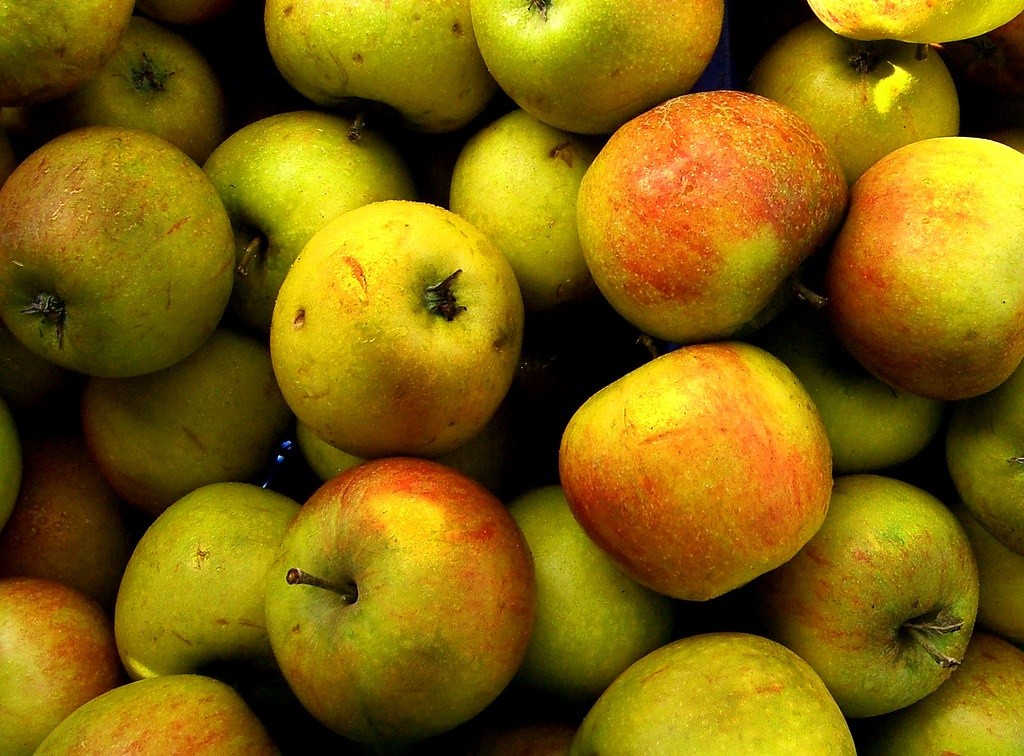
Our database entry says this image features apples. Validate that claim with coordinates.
[0,0,1024,756]
